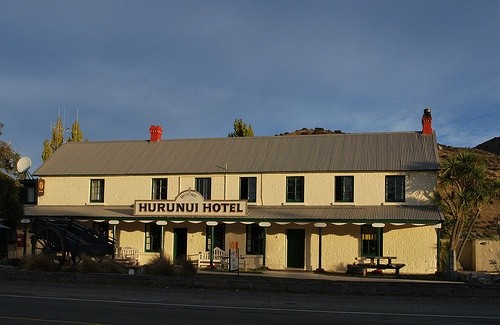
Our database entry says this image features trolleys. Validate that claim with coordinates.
[22,216,117,273]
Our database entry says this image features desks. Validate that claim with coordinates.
[365,256,397,269]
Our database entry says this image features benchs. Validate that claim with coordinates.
[376,264,405,276]
[357,264,386,277]
[198,247,229,270]
